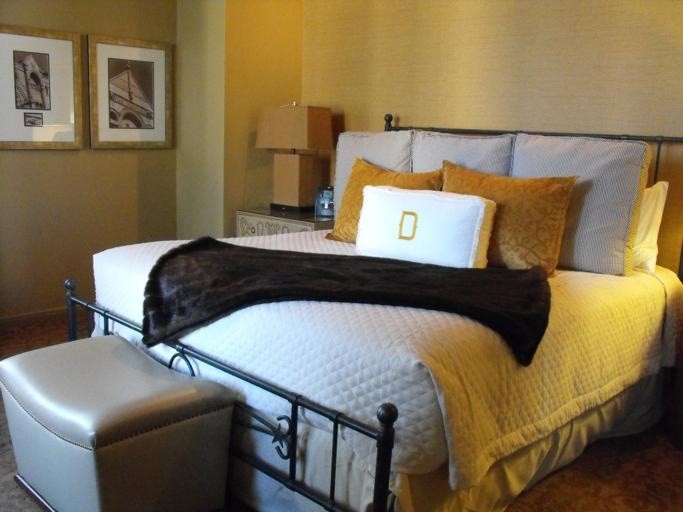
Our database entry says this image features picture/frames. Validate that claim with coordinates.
[87,33,176,152]
[0,22,90,152]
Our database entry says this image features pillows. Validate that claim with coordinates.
[352,183,497,270]
[632,178,668,278]
[324,156,443,245]
[508,130,654,279]
[408,124,514,177]
[333,128,411,229]
[436,156,579,279]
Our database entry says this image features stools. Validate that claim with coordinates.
[1,332,240,510]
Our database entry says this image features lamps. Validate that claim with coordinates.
[254,100,343,213]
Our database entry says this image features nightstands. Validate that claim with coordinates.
[234,205,332,236]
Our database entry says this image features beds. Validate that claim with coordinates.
[64,111,682,511]
